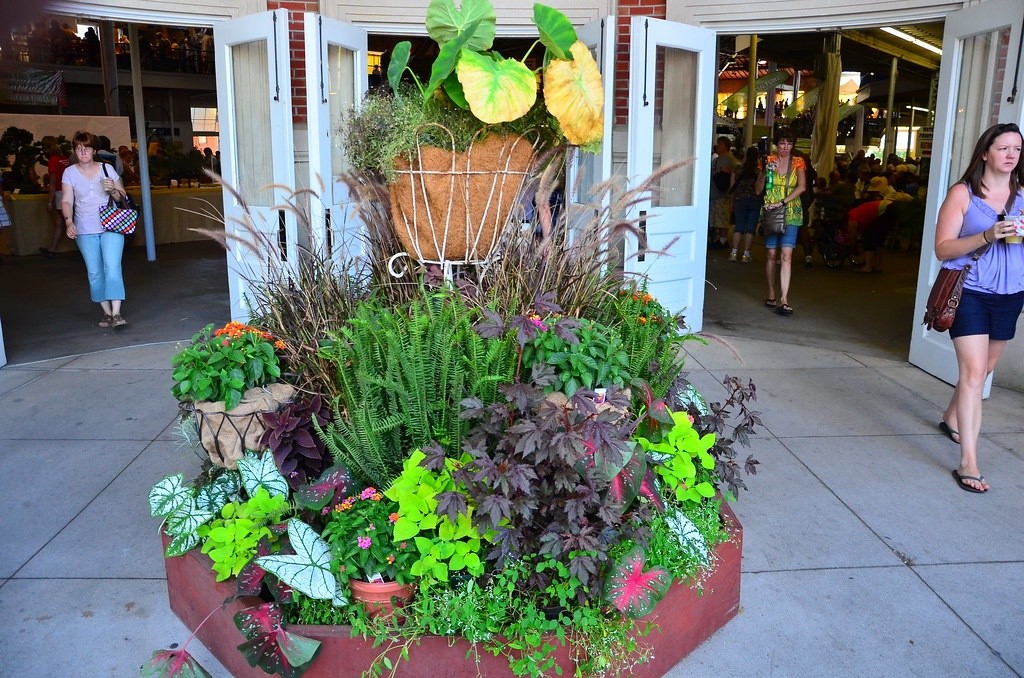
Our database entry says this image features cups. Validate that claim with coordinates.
[1004,215,1024,243]
[101,177,112,190]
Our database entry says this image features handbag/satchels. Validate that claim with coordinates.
[100,190,140,235]
[763,206,786,237]
[922,266,966,336]
[714,171,730,192]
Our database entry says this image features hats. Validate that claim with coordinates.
[867,176,888,191]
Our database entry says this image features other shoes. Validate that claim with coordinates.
[709,238,729,251]
[805,256,813,266]
[39,246,54,260]
[853,266,878,274]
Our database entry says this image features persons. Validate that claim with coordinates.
[61,133,128,329]
[755,132,807,314]
[758,96,789,121]
[518,167,566,261]
[0,20,215,76]
[0,197,13,259]
[791,104,869,139]
[3,136,222,257]
[709,136,928,274]
[725,98,738,119]
[934,124,1024,494]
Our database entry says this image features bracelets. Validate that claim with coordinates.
[759,179,764,183]
[984,230,992,245]
[64,217,72,220]
[112,190,116,195]
[781,199,787,207]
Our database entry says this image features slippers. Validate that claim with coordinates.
[951,467,988,495]
[776,304,794,315]
[937,419,961,446]
[766,296,777,307]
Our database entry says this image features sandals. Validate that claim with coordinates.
[112,314,126,328]
[99,314,112,327]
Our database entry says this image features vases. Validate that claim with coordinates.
[189,383,292,467]
[349,579,416,624]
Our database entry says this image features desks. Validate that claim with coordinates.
[0,181,225,258]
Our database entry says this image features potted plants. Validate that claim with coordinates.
[338,0,606,263]
[348,547,662,677]
[523,315,634,435]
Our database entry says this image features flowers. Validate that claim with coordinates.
[322,490,425,585]
[168,320,287,408]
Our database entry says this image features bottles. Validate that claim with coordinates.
[766,163,774,189]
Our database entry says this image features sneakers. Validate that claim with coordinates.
[741,254,752,263]
[728,252,737,262]
